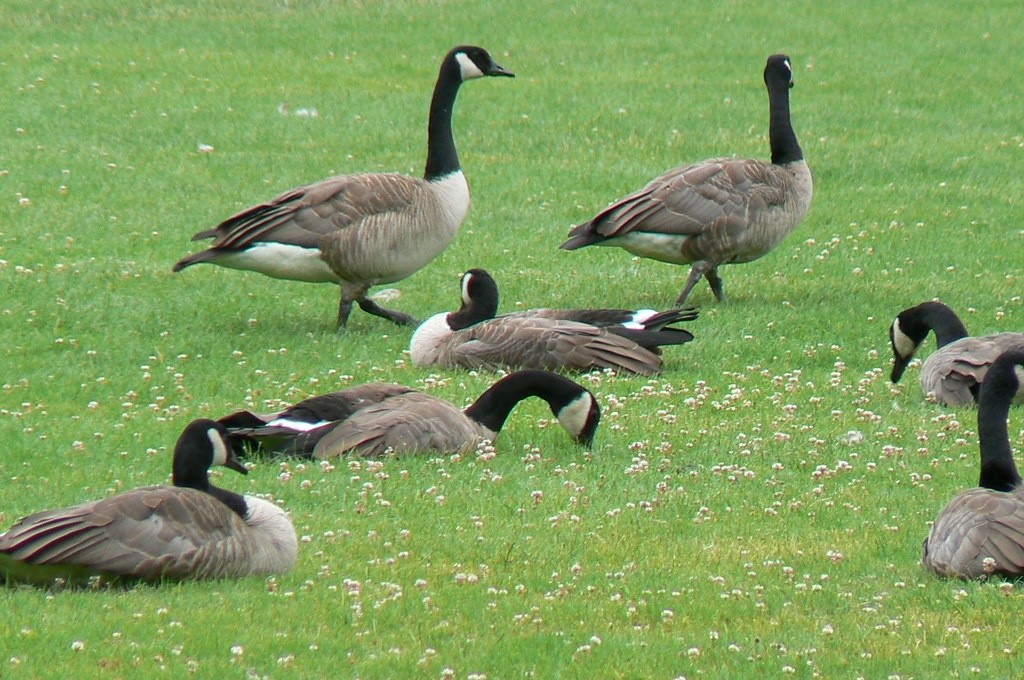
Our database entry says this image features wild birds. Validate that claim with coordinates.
[922,352,1024,584]
[408,267,702,380]
[214,370,602,462]
[0,418,298,588]
[889,300,1024,412]
[555,53,814,308]
[171,45,515,335]
